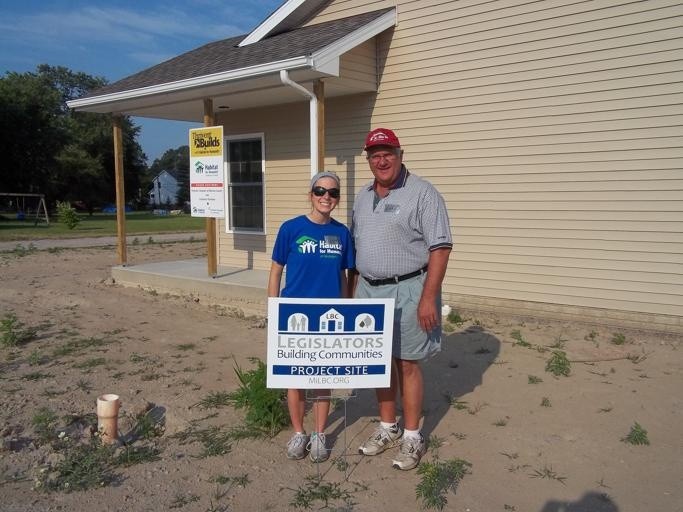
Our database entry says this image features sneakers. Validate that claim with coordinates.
[358,424,404,457]
[286,432,309,460]
[309,433,329,463]
[393,435,426,469]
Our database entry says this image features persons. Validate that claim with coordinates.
[347,128,454,471]
[266,171,359,463]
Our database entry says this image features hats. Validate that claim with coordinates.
[363,128,400,151]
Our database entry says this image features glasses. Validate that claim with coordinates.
[309,185,341,199]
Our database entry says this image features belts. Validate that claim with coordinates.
[365,266,428,286]
